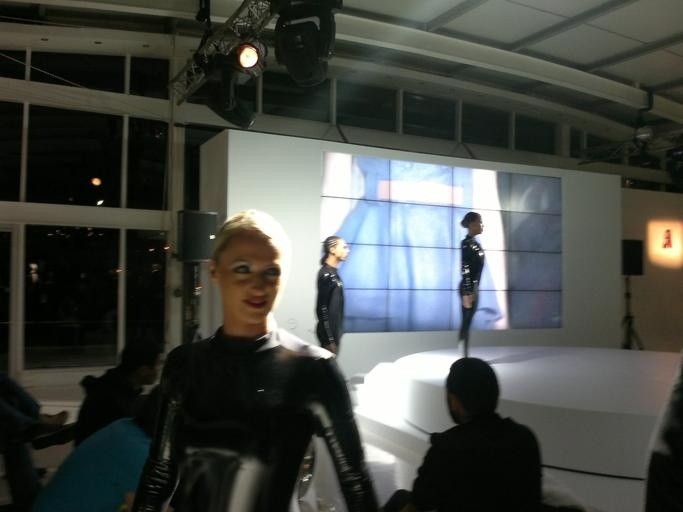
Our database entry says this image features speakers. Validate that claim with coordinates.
[621,237,644,275]
[175,209,219,259]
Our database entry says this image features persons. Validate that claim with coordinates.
[643,353,682,511]
[380,357,543,509]
[0,333,164,510]
[454,211,486,359]
[316,236,351,360]
[126,208,380,512]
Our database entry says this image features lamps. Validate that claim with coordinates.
[200,56,256,131]
[231,42,259,68]
[272,2,336,88]
[632,117,653,142]
